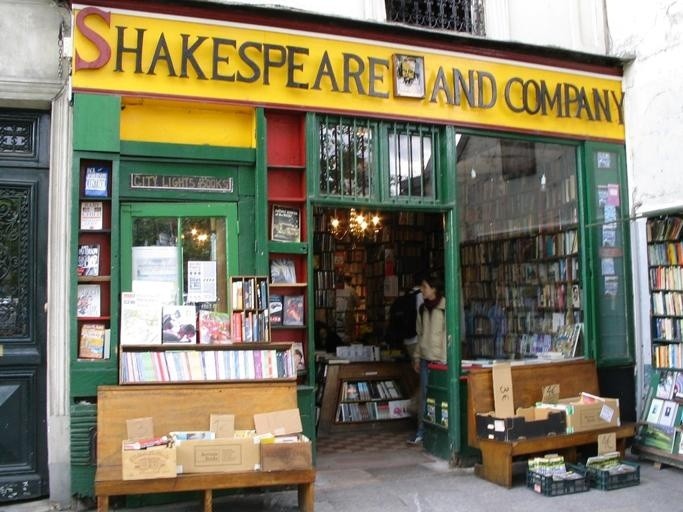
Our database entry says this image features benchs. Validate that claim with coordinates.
[94,382,317,511]
[465,356,639,490]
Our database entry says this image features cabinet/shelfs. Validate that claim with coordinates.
[645,207,683,372]
[455,146,628,359]
[72,149,120,369]
[263,105,311,381]
[311,204,445,352]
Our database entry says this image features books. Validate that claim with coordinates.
[83,166,110,198]
[459,175,584,366]
[76,284,102,317]
[122,404,310,450]
[282,294,305,325]
[587,451,621,470]
[269,257,296,283]
[77,243,101,277]
[530,453,582,482]
[311,210,447,426]
[636,213,683,455]
[271,203,302,243]
[80,201,103,231]
[78,324,105,359]
[120,260,296,388]
[291,342,305,370]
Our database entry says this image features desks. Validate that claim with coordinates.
[315,349,422,440]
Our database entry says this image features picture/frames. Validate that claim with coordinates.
[391,52,427,100]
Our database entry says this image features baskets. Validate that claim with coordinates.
[524,461,591,497]
[587,460,640,491]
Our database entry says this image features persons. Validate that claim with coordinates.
[396,58,424,98]
[406,275,447,447]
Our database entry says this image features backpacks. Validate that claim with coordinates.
[386,287,422,341]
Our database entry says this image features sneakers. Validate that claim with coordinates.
[406,436,423,445]
